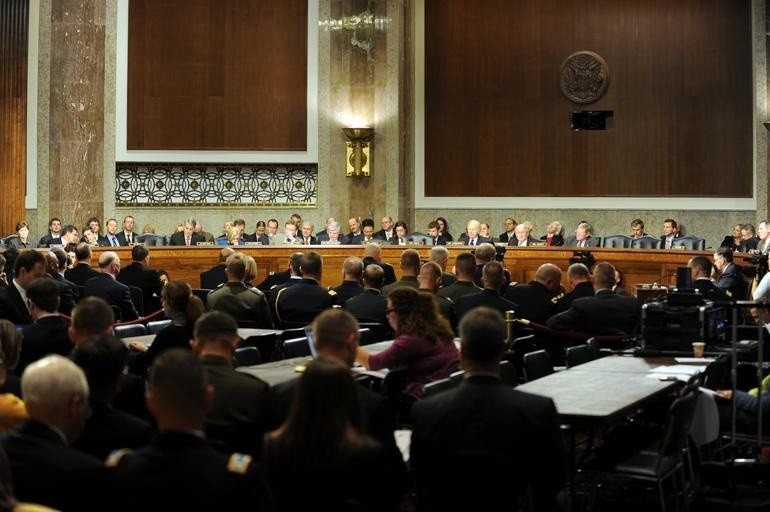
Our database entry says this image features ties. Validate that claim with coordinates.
[469,238,475,245]
[127,233,132,246]
[185,236,192,246]
[577,240,584,247]
[305,238,309,245]
[112,237,119,247]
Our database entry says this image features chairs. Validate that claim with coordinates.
[0,225,770,511]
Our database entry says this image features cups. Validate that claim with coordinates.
[692,342,706,357]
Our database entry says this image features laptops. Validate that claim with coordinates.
[304,326,363,367]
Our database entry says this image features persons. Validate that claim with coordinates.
[0,213,770,512]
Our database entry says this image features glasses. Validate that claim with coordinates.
[383,307,398,315]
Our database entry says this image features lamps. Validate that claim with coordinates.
[342,121,376,180]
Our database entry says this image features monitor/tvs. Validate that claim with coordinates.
[704,306,733,352]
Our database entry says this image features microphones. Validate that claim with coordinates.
[653,272,680,285]
